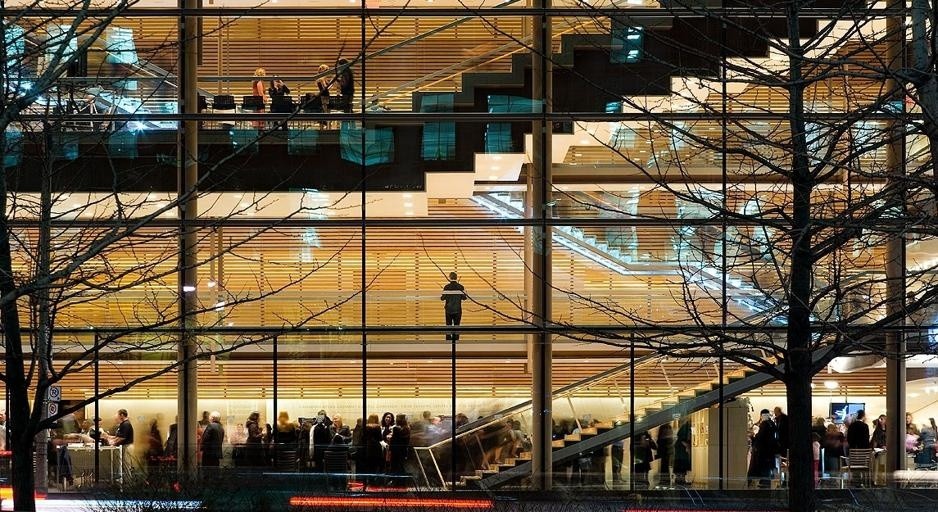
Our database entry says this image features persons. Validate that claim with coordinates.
[552,413,692,489]
[251,67,268,128]
[748,405,938,490]
[362,410,530,491]
[269,77,290,130]
[335,59,359,132]
[315,65,336,131]
[196,407,353,484]
[1,402,177,487]
[440,265,468,340]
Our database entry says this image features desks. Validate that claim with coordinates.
[56,443,124,492]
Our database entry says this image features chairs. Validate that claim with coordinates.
[327,97,352,130]
[298,94,318,133]
[238,93,266,127]
[839,447,874,489]
[210,91,237,128]
[197,94,208,123]
[270,95,295,131]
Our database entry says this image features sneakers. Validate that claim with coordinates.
[752,477,787,489]
[612,478,693,489]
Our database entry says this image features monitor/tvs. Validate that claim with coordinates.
[829,402,865,425]
